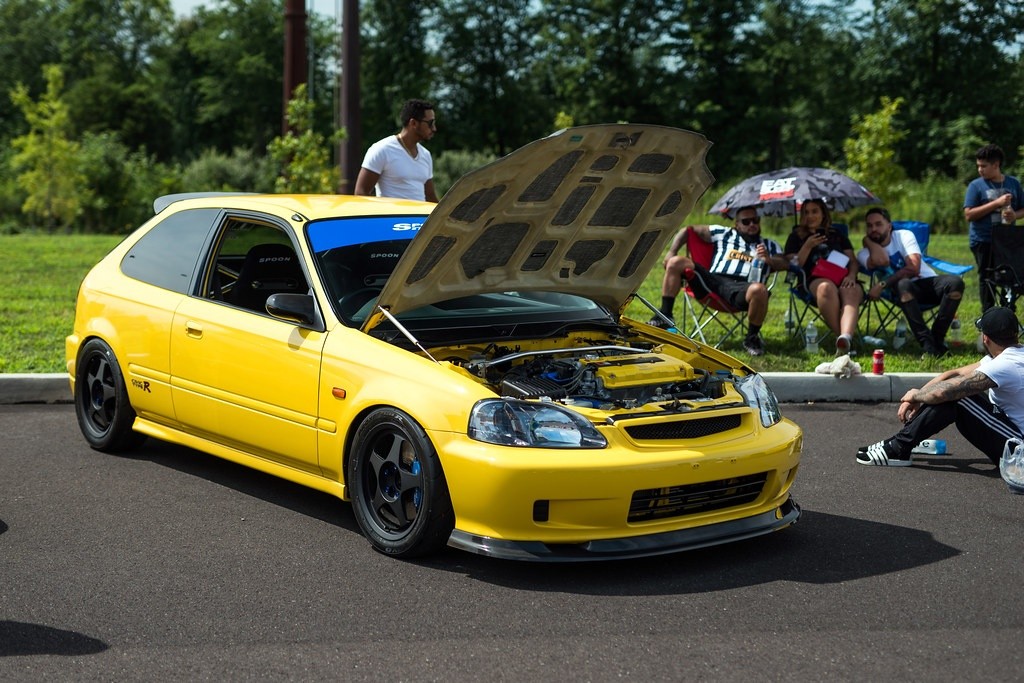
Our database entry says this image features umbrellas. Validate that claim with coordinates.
[708,167,881,224]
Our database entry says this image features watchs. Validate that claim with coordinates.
[880,281,886,288]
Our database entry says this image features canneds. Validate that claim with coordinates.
[874,349,884,375]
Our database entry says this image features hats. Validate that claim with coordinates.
[974,307,1019,347]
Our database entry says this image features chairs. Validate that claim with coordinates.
[663,225,783,351]
[856,217,976,344]
[984,217,1024,343]
[233,242,308,317]
[787,220,868,351]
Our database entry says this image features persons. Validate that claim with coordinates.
[646,206,785,356]
[855,306,1024,466]
[963,144,1024,313]
[785,198,864,358]
[354,99,439,203]
[858,207,965,359]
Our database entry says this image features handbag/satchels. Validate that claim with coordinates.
[999,438,1024,491]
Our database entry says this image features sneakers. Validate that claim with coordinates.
[856,437,912,466]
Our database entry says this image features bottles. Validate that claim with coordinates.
[893,314,907,349]
[912,440,946,455]
[807,320,818,352]
[864,336,885,346]
[786,254,799,283]
[748,252,764,282]
[950,315,961,346]
[784,307,795,333]
[1001,204,1010,224]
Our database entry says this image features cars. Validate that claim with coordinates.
[66,122,804,564]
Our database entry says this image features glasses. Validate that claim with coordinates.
[736,216,761,226]
[418,120,436,128]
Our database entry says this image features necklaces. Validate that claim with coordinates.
[990,176,1002,194]
[399,134,412,156]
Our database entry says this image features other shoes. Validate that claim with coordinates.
[835,335,851,357]
[648,315,675,328]
[742,335,765,357]
[919,345,952,360]
